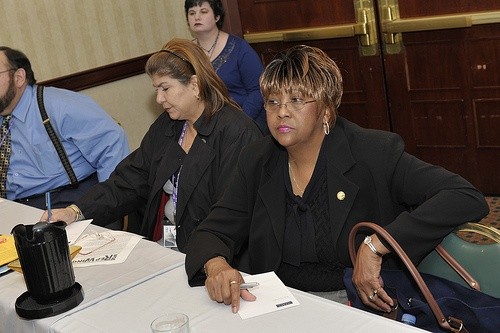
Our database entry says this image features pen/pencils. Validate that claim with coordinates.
[46,192,51,223]
[239,282,259,290]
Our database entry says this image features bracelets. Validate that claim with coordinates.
[65,205,81,223]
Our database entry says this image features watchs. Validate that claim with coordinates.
[363,236,384,257]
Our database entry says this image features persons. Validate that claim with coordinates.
[184,44,490,317]
[0,46,129,231]
[184,0,269,135]
[39,39,265,254]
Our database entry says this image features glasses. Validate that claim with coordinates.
[263,96,316,111]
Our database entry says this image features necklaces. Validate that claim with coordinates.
[196,29,220,60]
[288,154,304,198]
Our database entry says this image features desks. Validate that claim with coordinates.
[0,198,431,333]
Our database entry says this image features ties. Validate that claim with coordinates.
[0,114,12,199]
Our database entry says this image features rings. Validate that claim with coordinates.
[373,287,383,295]
[229,281,239,285]
[367,293,376,302]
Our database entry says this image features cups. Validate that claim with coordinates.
[150,313,190,333]
[11,220,75,304]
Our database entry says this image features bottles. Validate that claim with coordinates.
[401,313,417,325]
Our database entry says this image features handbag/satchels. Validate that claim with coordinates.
[341,221,500,333]
